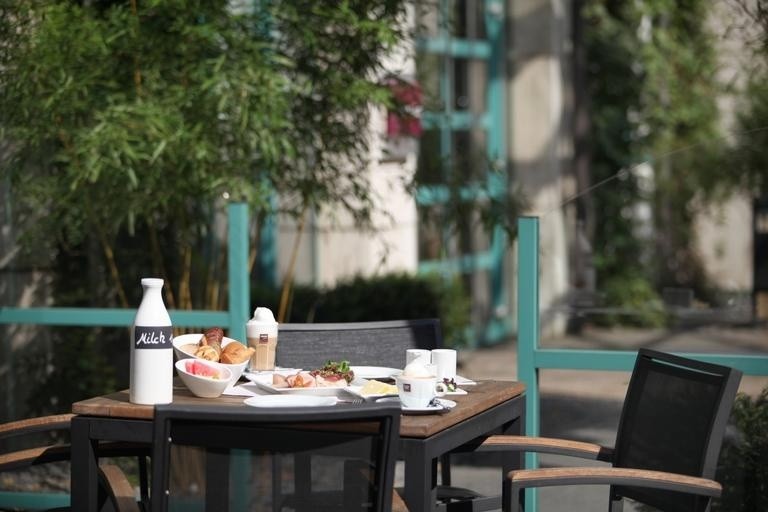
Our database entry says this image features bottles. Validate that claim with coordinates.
[128,275,175,406]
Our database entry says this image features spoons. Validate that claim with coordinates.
[431,399,450,412]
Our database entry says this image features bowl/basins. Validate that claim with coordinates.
[172,333,250,388]
[174,358,231,398]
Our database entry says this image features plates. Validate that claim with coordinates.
[375,397,456,414]
[348,366,404,381]
[343,377,398,399]
[431,381,468,398]
[243,395,337,409]
[453,374,476,386]
[253,376,344,394]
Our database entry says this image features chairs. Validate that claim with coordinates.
[441,348,743,512]
[70,401,409,512]
[273,319,485,512]
[0,414,154,512]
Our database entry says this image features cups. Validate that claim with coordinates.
[405,349,431,368]
[396,373,447,409]
[431,348,456,380]
[245,324,277,374]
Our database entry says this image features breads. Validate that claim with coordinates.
[193,328,255,366]
